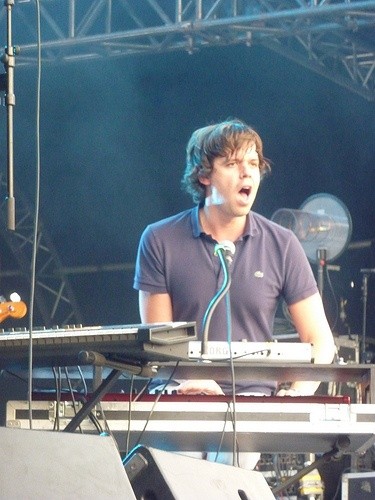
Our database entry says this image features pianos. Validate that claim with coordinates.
[0,321,198,362]
[32,390,351,404]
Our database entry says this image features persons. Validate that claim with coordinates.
[132,117,336,470]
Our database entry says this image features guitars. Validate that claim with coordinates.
[0,291,28,324]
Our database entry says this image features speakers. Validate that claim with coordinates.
[0,426,138,500]
[123,444,277,500]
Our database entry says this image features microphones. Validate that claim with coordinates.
[219,240,236,274]
